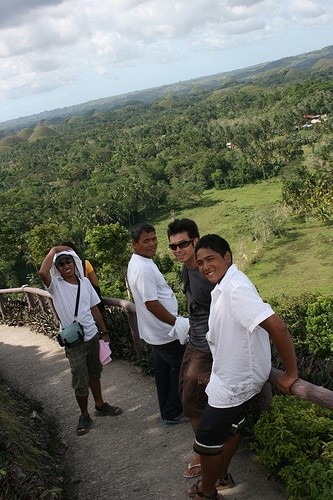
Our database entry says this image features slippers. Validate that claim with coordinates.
[184,459,203,478]
[165,416,191,425]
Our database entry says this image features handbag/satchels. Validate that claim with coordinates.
[56,320,85,347]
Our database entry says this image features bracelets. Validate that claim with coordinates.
[100,330,108,335]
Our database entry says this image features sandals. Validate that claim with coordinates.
[76,415,93,435]
[93,402,122,415]
[193,488,225,500]
[188,473,234,497]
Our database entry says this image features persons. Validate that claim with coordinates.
[39,246,122,434]
[188,234,298,500]
[125,224,192,424]
[62,243,105,336]
[165,218,223,478]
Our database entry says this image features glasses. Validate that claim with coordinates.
[167,238,195,250]
[59,258,72,267]
